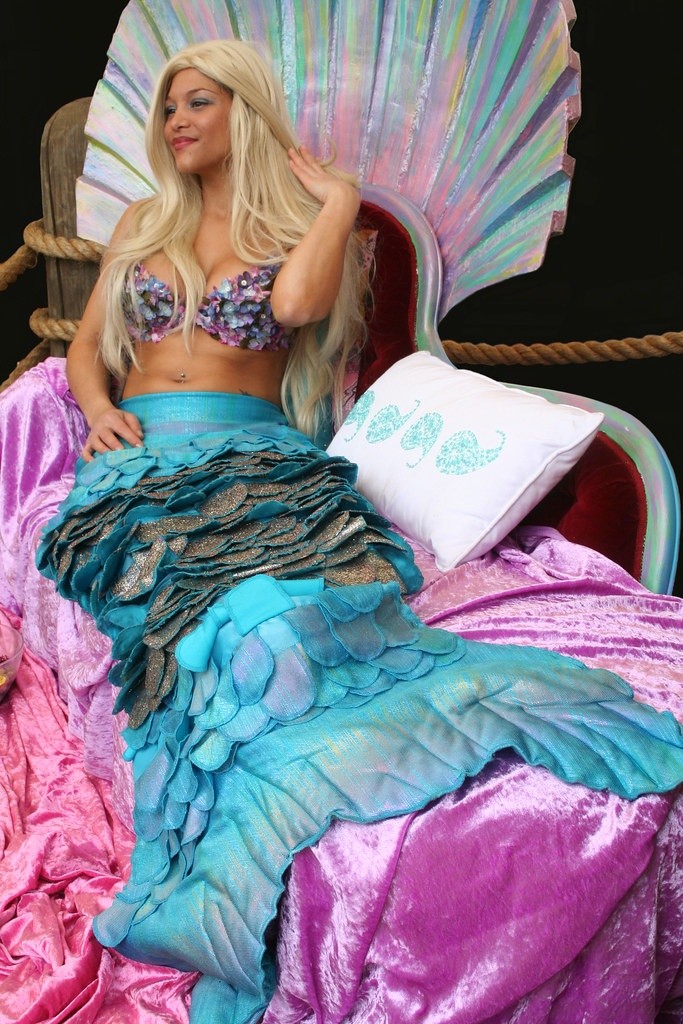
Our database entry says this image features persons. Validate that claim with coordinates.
[37,40,683,1024]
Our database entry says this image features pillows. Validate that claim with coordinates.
[322,352,603,572]
